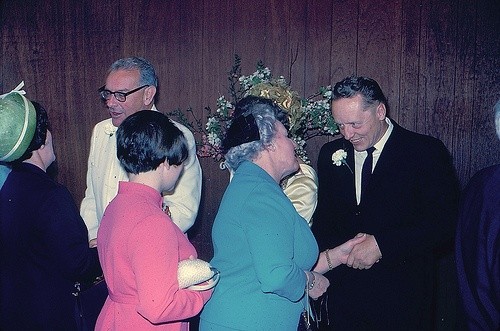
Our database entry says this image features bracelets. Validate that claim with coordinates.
[306,272,315,290]
[326,250,334,271]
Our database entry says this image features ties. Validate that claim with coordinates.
[360,146,376,201]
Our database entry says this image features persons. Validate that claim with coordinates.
[0,81,106,331]
[198,94,377,331]
[78,57,201,331]
[313,75,454,331]
[93,109,218,331]
[456,102,500,331]
[223,96,319,230]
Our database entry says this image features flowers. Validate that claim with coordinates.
[332,149,353,174]
[166,55,340,164]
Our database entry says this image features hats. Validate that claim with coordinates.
[0,80,37,162]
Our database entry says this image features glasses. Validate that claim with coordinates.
[97,85,149,102]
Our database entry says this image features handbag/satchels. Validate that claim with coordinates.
[176,258,220,291]
[65,247,105,299]
